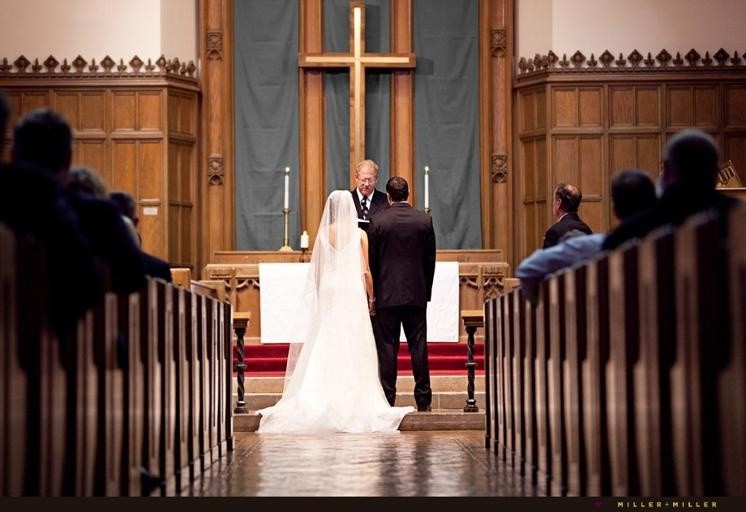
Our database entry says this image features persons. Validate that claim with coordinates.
[281,190,376,416]
[368,176,436,411]
[350,159,387,228]
[1,95,174,330]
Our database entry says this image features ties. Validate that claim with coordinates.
[360,195,368,220]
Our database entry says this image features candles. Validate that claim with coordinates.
[300,231,310,249]
[284,167,289,210]
[424,165,430,209]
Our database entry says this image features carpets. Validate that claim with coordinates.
[230,340,485,377]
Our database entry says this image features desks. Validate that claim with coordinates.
[230,310,252,415]
[460,310,487,413]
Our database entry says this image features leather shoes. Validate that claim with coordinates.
[416,402,431,412]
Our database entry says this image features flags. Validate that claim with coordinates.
[514,130,745,303]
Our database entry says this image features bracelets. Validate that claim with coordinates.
[368,297,376,302]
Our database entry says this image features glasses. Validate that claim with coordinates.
[361,179,376,184]
